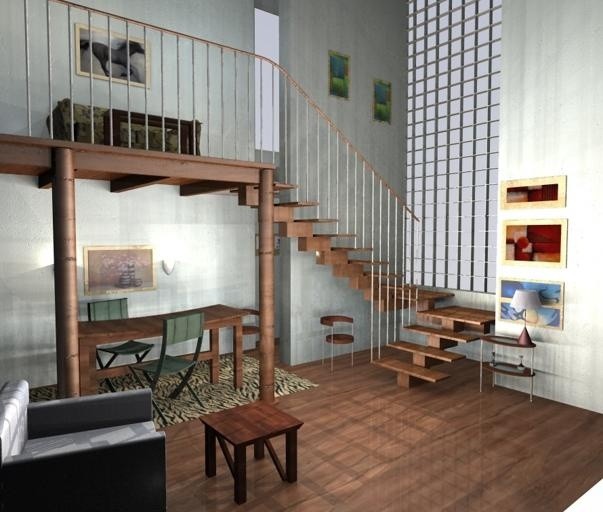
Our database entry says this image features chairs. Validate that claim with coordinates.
[318,314,355,373]
[87,297,207,410]
[0,377,167,511]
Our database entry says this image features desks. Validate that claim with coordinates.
[75,303,250,396]
[100,109,196,156]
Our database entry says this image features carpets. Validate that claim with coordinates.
[26,351,318,430]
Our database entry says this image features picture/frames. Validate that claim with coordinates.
[82,243,156,295]
[497,175,568,331]
[328,48,393,127]
[73,23,151,91]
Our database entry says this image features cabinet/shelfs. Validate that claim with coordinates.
[479,333,537,403]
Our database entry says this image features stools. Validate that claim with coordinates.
[197,399,303,505]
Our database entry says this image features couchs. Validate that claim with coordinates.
[45,98,203,155]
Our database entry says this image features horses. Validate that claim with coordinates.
[80,39,145,77]
